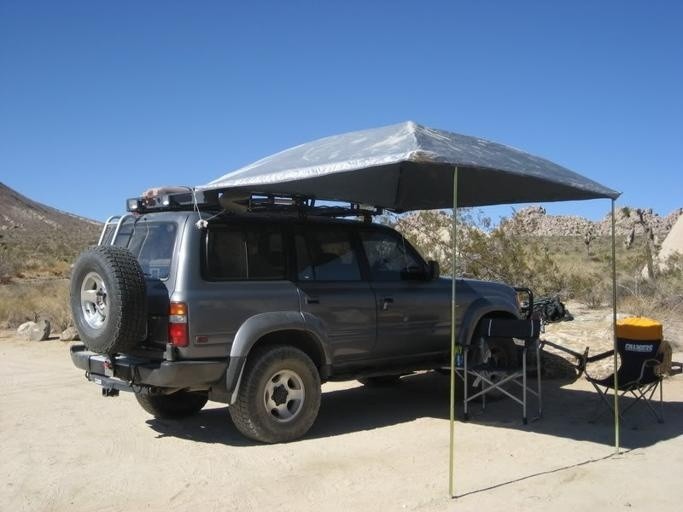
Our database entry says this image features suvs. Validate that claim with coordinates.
[71,188,533,442]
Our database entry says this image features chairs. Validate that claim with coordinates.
[583,318,664,430]
[463,318,542,425]
